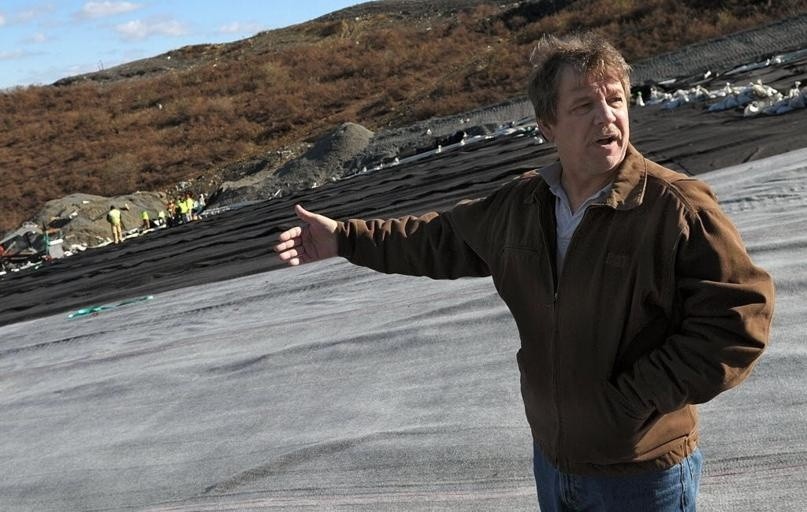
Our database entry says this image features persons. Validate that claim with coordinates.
[273,28,775,510]
[141,192,209,230]
[106,204,125,246]
